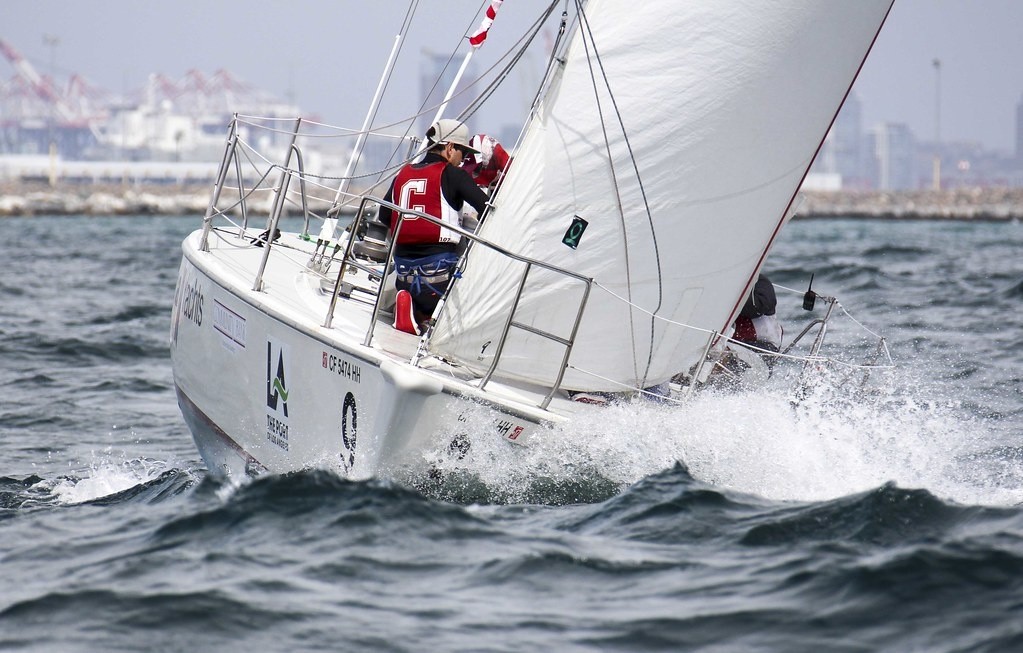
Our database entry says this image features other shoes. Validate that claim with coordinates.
[392,290,421,336]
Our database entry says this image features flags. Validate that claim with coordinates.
[470,0,503,49]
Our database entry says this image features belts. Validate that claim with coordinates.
[397,271,450,283]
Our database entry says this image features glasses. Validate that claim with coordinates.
[454,146,468,158]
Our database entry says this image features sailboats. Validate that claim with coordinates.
[168,0,895,472]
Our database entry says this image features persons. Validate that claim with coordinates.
[379,117,490,335]
[562,273,782,405]
[460,134,513,196]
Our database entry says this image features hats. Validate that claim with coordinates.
[430,119,480,154]
